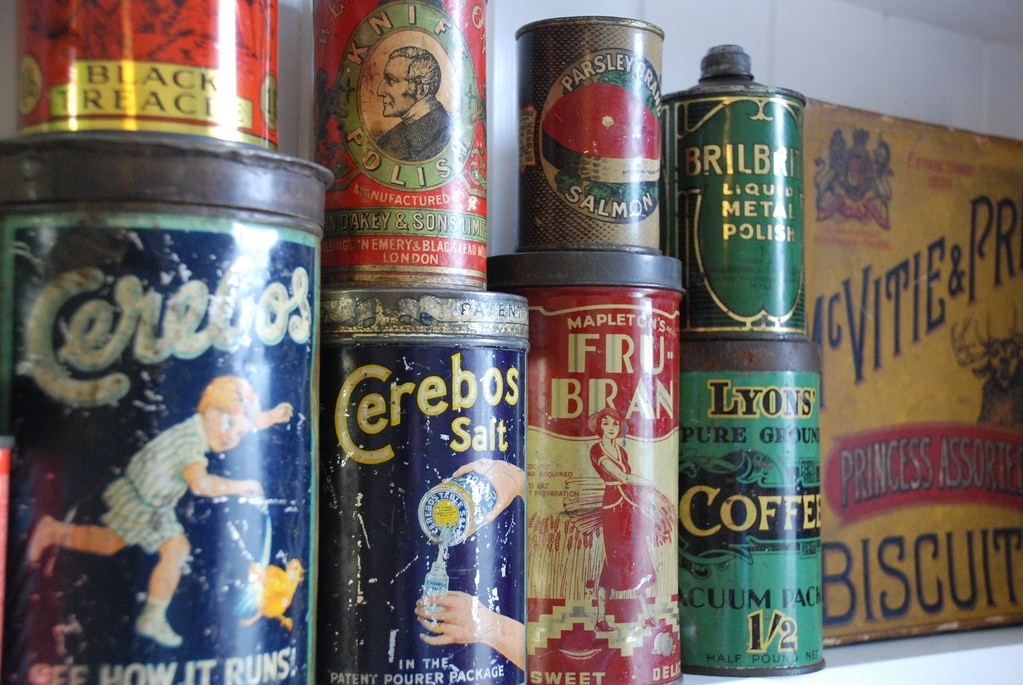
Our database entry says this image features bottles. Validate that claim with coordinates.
[0,131,314,685]
[17,0,278,151]
[514,15,665,255]
[313,0,486,292]
[658,44,808,339]
[313,289,525,685]
